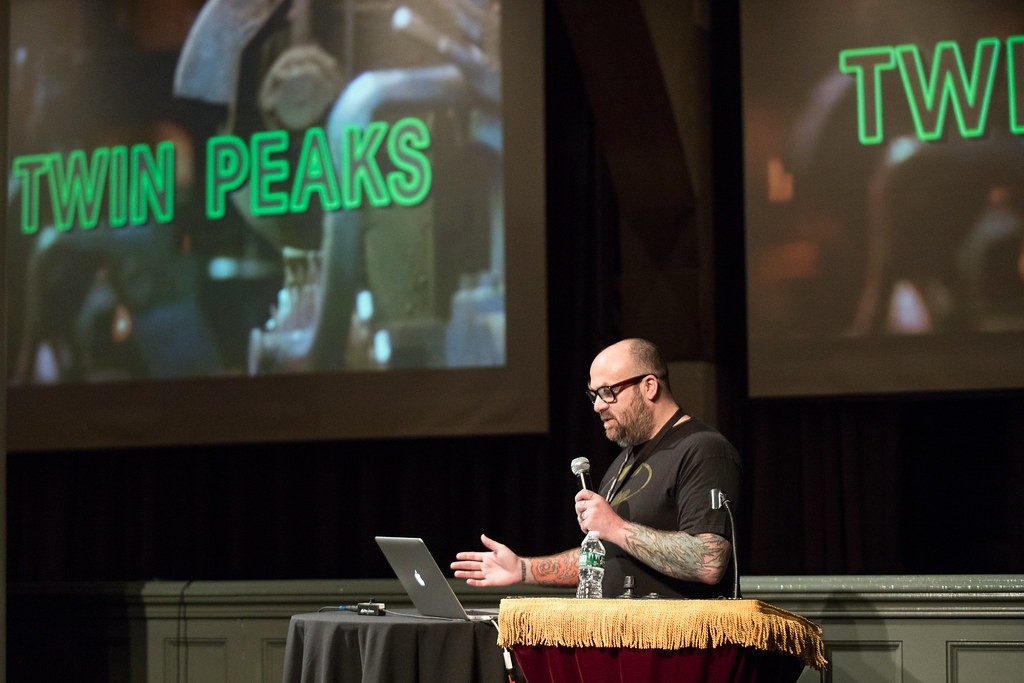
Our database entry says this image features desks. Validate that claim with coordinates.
[284,607,510,683]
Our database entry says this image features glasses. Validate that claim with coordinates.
[587,374,658,404]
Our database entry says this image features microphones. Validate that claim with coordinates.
[570,457,594,494]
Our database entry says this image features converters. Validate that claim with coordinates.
[356,603,386,616]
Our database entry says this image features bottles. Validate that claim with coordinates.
[577,531,606,598]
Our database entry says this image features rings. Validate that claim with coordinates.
[579,512,585,521]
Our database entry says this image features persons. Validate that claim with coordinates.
[451,337,742,602]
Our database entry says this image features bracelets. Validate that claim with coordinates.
[520,558,527,582]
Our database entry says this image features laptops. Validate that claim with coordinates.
[375,537,499,621]
